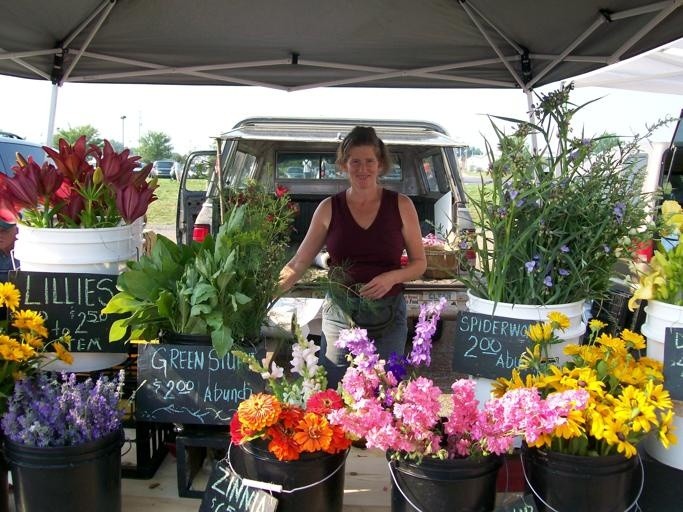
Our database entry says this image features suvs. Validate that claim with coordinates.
[0,130,59,281]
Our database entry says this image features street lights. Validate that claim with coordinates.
[120,114,126,147]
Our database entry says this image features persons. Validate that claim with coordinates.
[0,212,24,282]
[270,126,428,392]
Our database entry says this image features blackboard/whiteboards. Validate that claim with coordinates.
[663,327,683,400]
[6,270,132,354]
[198,460,278,512]
[452,310,543,379]
[135,342,266,426]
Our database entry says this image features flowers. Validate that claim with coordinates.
[0,82,683,463]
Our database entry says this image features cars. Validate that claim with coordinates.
[282,166,303,179]
[129,160,209,180]
[315,156,433,180]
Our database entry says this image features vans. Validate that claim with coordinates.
[174,116,477,338]
[609,107,683,293]
